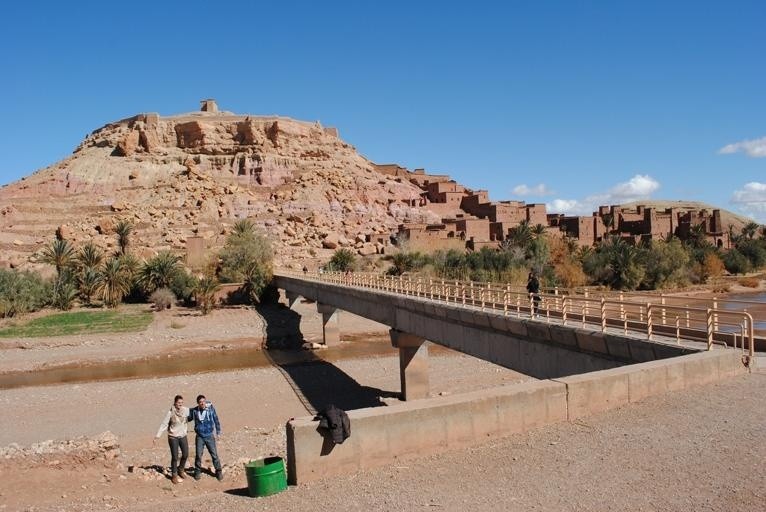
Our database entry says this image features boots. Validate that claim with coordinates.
[195,473,201,480]
[216,472,223,481]
[172,472,188,483]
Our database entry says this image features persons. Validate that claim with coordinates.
[185,395,223,481]
[151,393,190,486]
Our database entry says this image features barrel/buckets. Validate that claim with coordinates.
[244,457,289,496]
